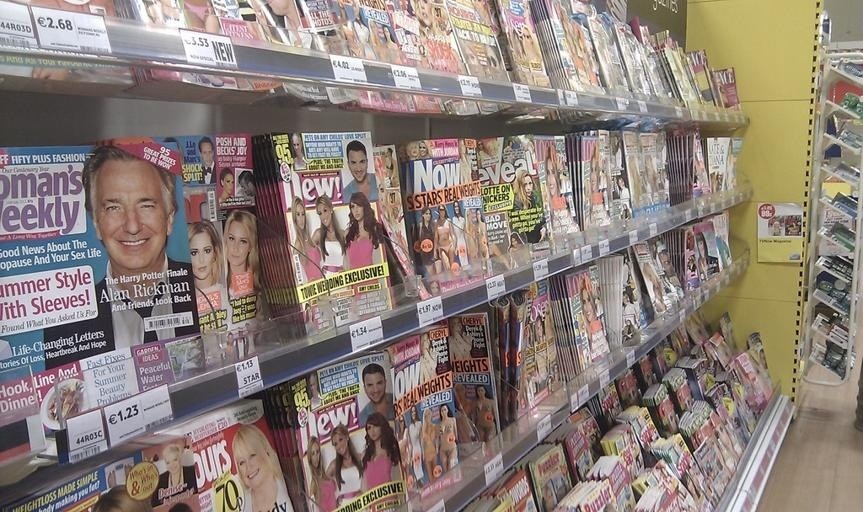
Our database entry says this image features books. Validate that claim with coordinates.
[0,1,780,511]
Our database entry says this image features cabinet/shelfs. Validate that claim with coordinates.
[799,49,861,387]
[1,2,795,512]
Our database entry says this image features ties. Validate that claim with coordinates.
[118,282,166,343]
